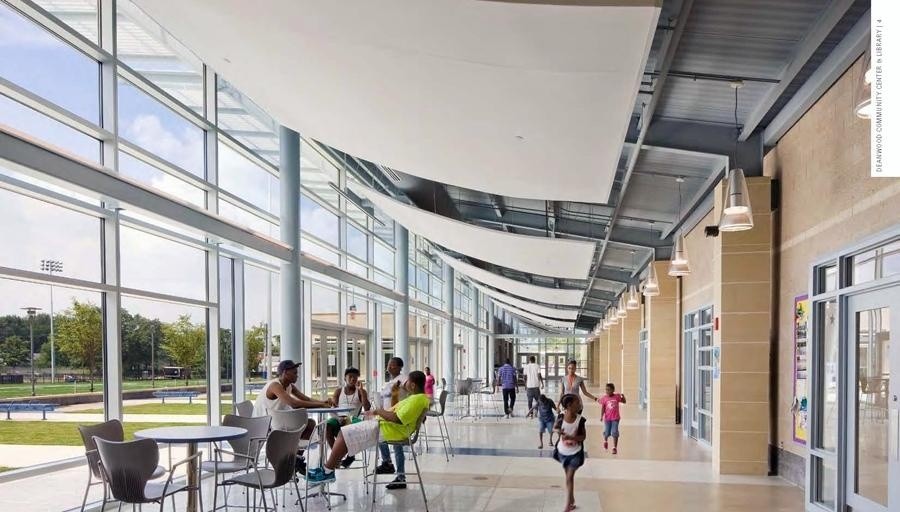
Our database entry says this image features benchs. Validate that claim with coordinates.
[152,392,200,406]
[0,401,61,420]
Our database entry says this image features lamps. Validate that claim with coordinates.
[853,36,871,120]
[615,266,628,318]
[667,175,692,276]
[627,248,639,309]
[643,220,660,296]
[718,79,753,232]
[584,304,618,342]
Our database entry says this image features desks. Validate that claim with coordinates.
[134,425,249,512]
[456,379,482,420]
[292,408,356,510]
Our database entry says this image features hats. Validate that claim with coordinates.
[277,359,302,373]
[345,366,360,375]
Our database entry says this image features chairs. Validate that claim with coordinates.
[266,408,328,507]
[299,420,330,511]
[475,378,501,421]
[91,436,203,512]
[77,419,165,512]
[235,400,254,417]
[201,414,272,512]
[441,377,480,420]
[418,390,455,463]
[366,408,428,512]
[213,424,306,512]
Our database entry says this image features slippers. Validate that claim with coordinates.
[570,504,576,510]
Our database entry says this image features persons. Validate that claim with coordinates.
[325,368,371,469]
[496,358,519,419]
[557,358,599,415]
[523,356,544,419]
[424,366,435,397]
[372,357,408,489]
[525,394,561,449]
[302,371,431,483]
[253,359,331,487]
[552,394,587,511]
[598,383,627,454]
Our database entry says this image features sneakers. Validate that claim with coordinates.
[611,447,617,454]
[295,458,307,475]
[342,456,355,468]
[504,415,509,418]
[304,467,336,484]
[603,442,608,449]
[386,477,407,489]
[373,461,395,474]
[509,412,515,418]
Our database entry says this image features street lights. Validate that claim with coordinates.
[40,259,63,384]
[20,307,41,396]
